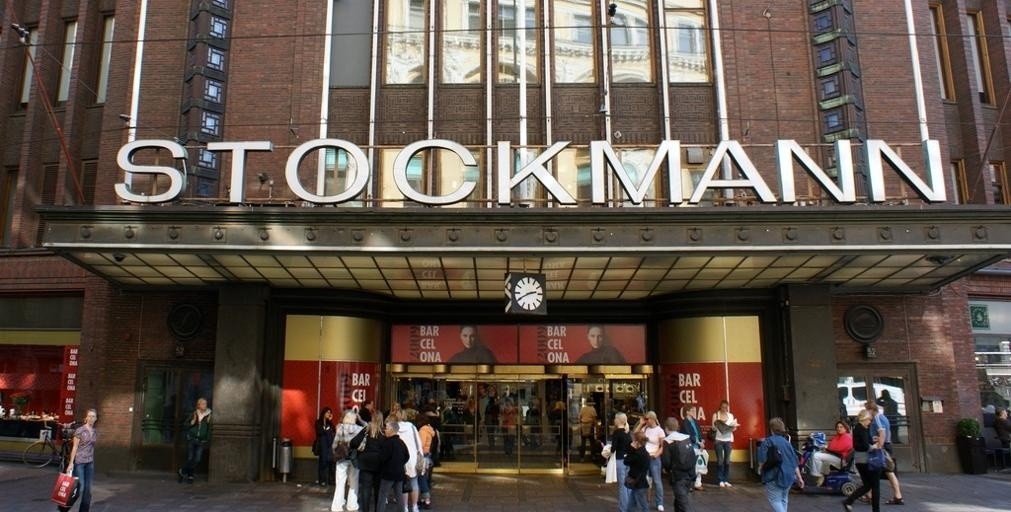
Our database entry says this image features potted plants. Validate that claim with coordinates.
[953,417,989,475]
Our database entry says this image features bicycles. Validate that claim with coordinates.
[22,414,84,473]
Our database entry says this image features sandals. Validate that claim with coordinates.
[885,496,905,505]
[841,493,871,512]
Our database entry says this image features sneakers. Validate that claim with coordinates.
[719,482,732,488]
[329,502,358,512]
[399,500,431,512]
[690,486,704,493]
[656,503,664,511]
[175,470,194,485]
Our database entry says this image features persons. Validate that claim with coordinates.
[757,400,906,512]
[577,397,709,510]
[439,398,567,463]
[711,399,736,487]
[573,323,627,366]
[993,405,1011,448]
[445,324,497,366]
[176,397,213,484]
[316,388,440,511]
[57,407,98,512]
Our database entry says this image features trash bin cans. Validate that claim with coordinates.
[272,438,293,484]
[749,438,765,476]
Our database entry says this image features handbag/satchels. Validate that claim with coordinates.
[430,429,442,466]
[762,438,784,476]
[416,452,424,474]
[867,449,884,470]
[707,411,718,443]
[693,448,709,476]
[311,438,319,457]
[50,465,78,509]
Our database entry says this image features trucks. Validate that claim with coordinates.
[837,381,907,425]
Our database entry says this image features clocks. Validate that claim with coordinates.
[510,272,548,317]
[969,304,991,331]
